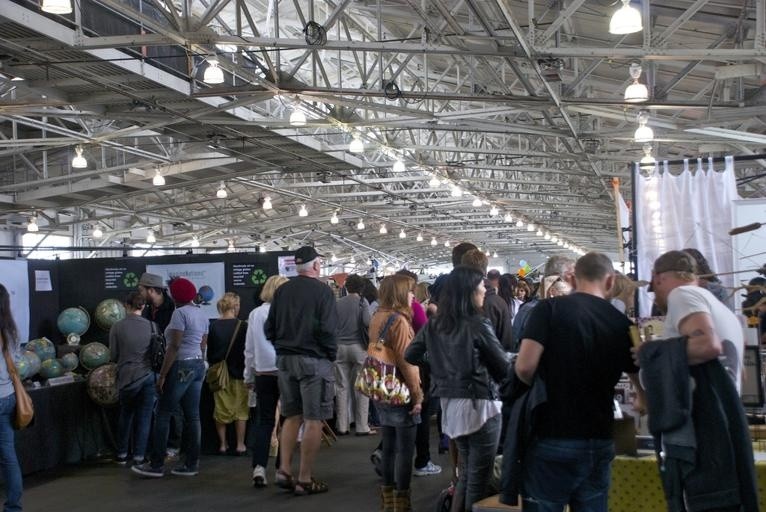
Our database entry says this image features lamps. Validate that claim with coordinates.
[203,51,225,85]
[633,109,654,142]
[262,193,272,209]
[72,143,88,169]
[640,144,656,167]
[348,132,364,153]
[151,167,166,186]
[392,154,407,173]
[290,98,306,127]
[624,60,650,104]
[429,174,441,188]
[216,179,227,198]
[40,1,73,15]
[298,198,308,217]
[608,0,645,38]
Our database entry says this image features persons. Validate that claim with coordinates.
[208,292,253,457]
[630,250,748,512]
[139,272,176,457]
[108,291,152,466]
[263,246,338,497]
[498,274,524,327]
[610,274,635,316]
[517,280,530,302]
[418,282,431,313]
[131,278,209,478]
[544,256,576,288]
[243,275,292,489]
[370,270,442,479]
[332,274,377,436]
[652,248,732,316]
[404,264,511,512]
[515,252,648,512]
[513,275,572,347]
[426,242,477,318]
[368,275,423,512]
[449,249,510,486]
[0,284,23,512]
[361,278,381,316]
[488,269,501,295]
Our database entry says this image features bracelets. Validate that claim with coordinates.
[158,374,168,378]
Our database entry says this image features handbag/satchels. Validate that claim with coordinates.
[1,338,35,431]
[150,323,165,374]
[357,295,369,352]
[353,313,412,406]
[205,320,242,393]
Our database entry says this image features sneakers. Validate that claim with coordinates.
[132,455,145,465]
[111,452,128,465]
[252,464,268,488]
[356,429,377,435]
[415,460,442,476]
[370,449,383,478]
[131,461,164,478]
[171,463,199,476]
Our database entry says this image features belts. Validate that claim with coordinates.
[184,355,202,360]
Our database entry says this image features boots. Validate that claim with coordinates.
[392,489,412,512]
[382,485,393,512]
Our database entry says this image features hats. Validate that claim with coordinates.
[647,250,698,291]
[294,246,325,265]
[169,278,196,304]
[137,272,163,288]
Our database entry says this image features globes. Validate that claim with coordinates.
[95,298,127,333]
[79,341,111,371]
[88,360,127,408]
[23,350,42,377]
[14,352,30,380]
[41,358,65,378]
[24,336,56,362]
[56,305,91,347]
[198,285,214,306]
[63,352,79,372]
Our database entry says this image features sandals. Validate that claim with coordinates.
[294,476,329,496]
[273,470,297,489]
